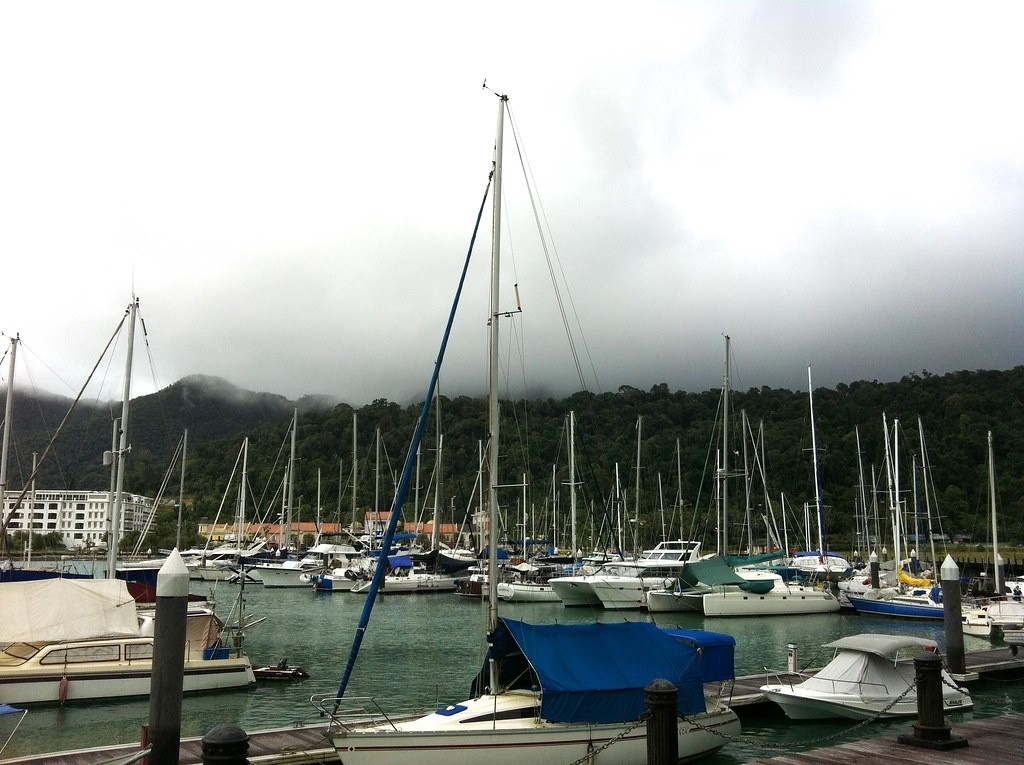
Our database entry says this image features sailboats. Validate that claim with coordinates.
[120,407,858,617]
[310,70,742,764]
[846,409,1024,641]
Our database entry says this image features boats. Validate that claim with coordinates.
[760,632,974,722]
[0,293,258,708]
[0,324,96,582]
[253,657,310,683]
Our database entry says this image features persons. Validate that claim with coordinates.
[1014,586,1022,602]
[818,551,823,564]
